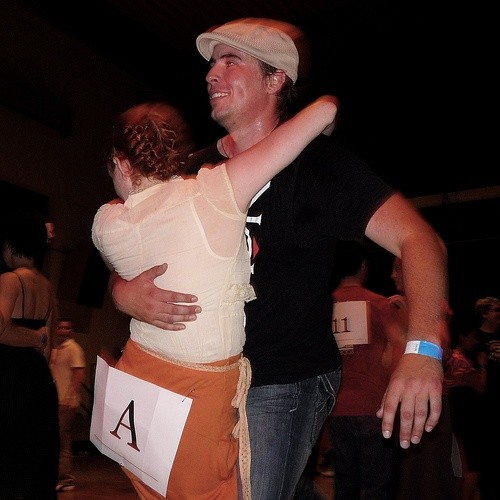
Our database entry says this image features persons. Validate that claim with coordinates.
[47,317,85,493]
[0,215,60,500]
[92,94,339,500]
[388,255,411,316]
[324,226,410,500]
[471,297,500,500]
[104,18,453,500]
[445,315,489,500]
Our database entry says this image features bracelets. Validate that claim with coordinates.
[403,340,443,361]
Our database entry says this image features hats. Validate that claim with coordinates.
[195,23,299,84]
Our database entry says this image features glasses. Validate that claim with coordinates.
[485,308,499,313]
